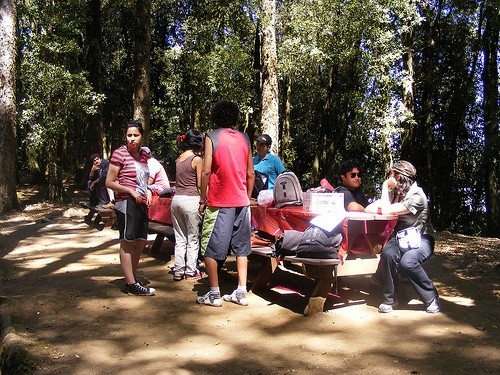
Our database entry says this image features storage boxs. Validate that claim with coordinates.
[303,192,344,213]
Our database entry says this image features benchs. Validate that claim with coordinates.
[148,222,175,259]
[252,248,341,310]
[83,202,111,232]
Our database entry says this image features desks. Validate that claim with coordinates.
[148,194,395,305]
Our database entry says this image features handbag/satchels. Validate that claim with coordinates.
[269,230,304,257]
[396,226,421,252]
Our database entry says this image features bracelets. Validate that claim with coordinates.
[93,182,94,184]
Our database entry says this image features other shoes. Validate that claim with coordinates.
[378,301,399,312]
[426,296,440,313]
[86,204,95,208]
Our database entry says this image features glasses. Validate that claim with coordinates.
[345,173,362,178]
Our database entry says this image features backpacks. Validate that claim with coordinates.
[296,224,344,258]
[273,170,303,208]
[251,170,268,199]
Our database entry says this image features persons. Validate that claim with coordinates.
[88,146,170,205]
[171,101,285,306]
[334,161,441,313]
[106,121,156,296]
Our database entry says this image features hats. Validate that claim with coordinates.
[256,134,272,145]
[390,160,417,178]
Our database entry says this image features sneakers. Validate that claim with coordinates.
[222,290,248,306]
[127,281,156,296]
[196,291,222,307]
[185,270,205,279]
[122,279,143,293]
[173,273,185,280]
[101,202,114,209]
